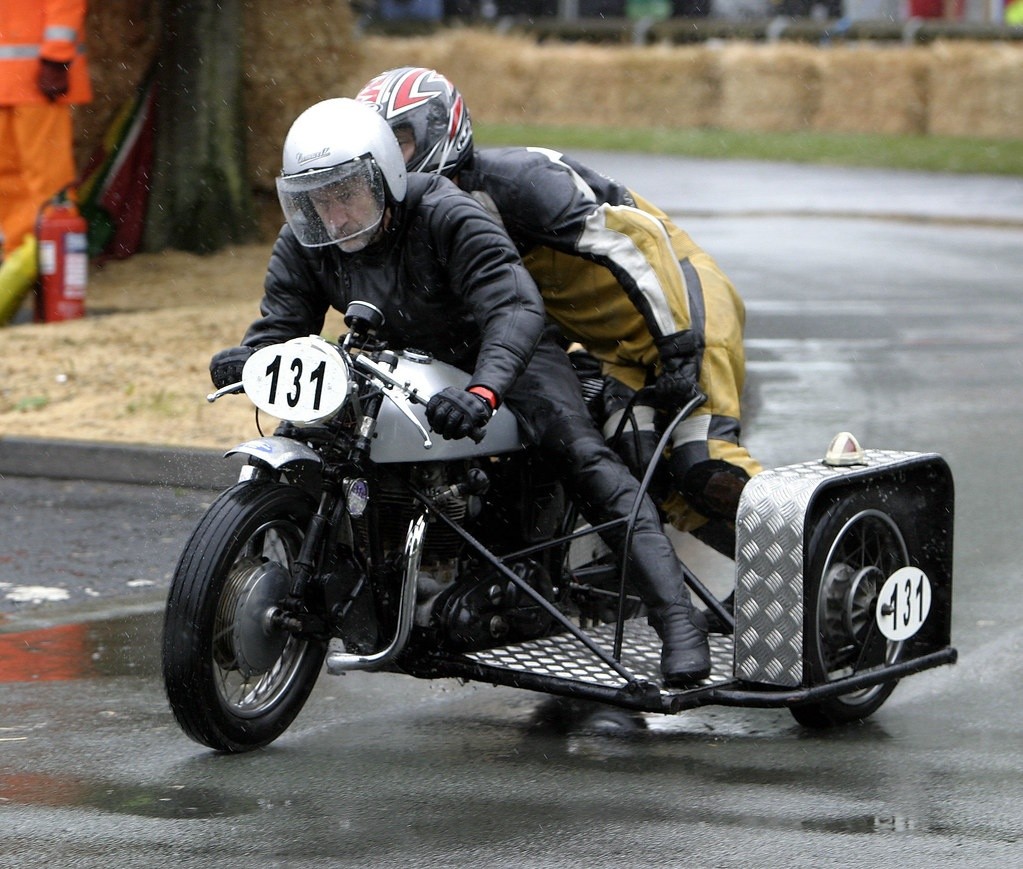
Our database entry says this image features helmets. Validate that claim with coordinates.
[282,98,406,203]
[356,68,472,178]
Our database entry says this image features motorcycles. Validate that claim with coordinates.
[163,298,959,758]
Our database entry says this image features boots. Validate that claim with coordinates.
[621,531,711,682]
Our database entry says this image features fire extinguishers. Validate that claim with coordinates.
[36,176,89,321]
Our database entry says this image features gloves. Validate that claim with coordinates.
[655,330,704,410]
[209,345,260,393]
[423,386,490,441]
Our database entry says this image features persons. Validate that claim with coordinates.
[210,93,710,683]
[352,66,769,532]
